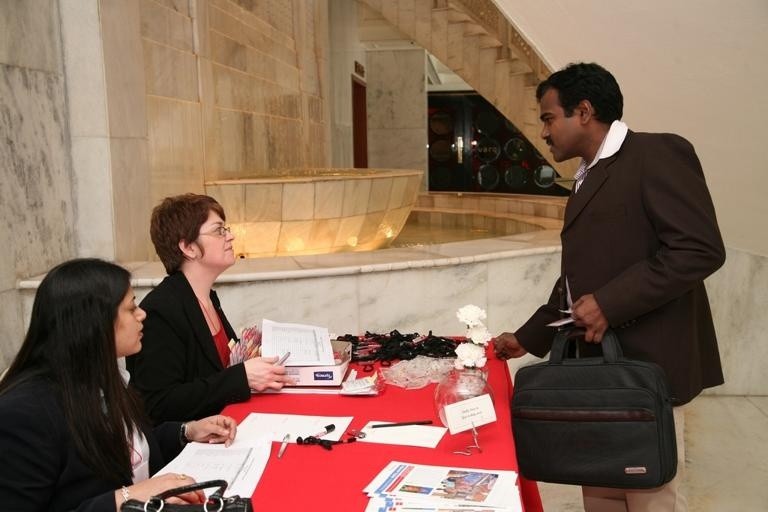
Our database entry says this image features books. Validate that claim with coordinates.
[284,342,354,389]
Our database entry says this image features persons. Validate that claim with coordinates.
[0,256,205,511]
[122,192,292,417]
[491,59,726,511]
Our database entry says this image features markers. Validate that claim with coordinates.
[297,424,334,444]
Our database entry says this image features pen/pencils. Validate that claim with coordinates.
[277,434,291,458]
[372,420,432,428]
[273,352,290,365]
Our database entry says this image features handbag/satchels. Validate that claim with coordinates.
[120,479,252,512]
[511,327,679,489]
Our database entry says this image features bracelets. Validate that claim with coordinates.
[117,483,130,505]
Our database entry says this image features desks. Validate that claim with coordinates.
[125,336,524,512]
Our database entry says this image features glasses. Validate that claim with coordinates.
[198,227,231,237]
